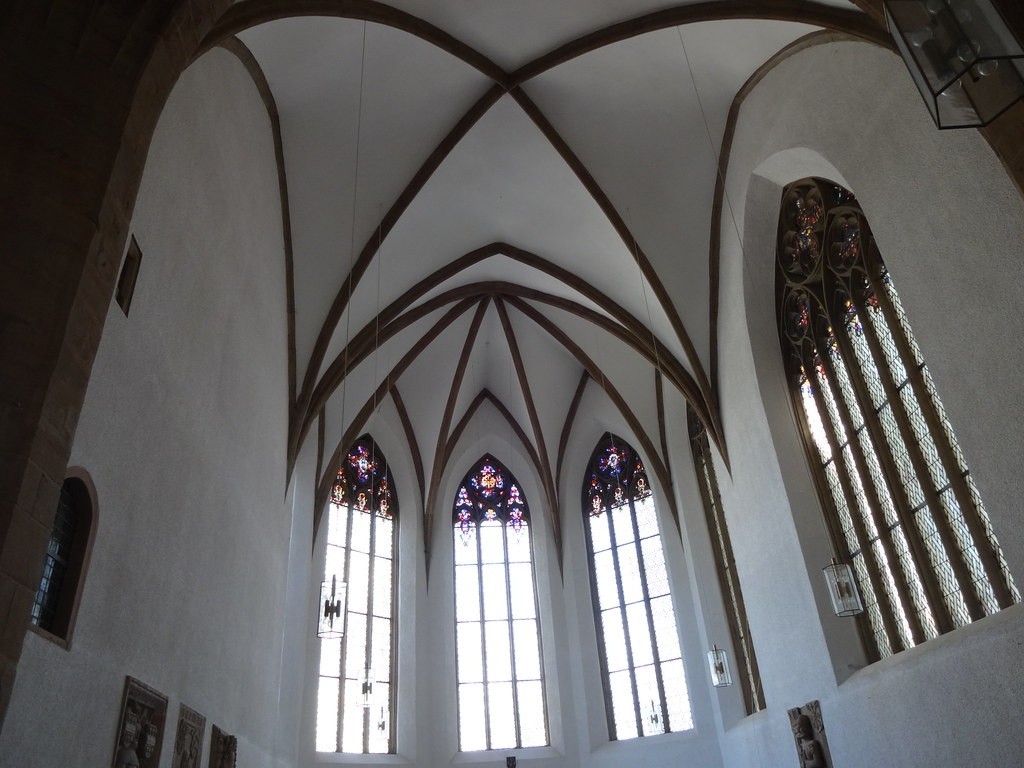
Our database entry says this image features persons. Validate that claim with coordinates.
[793,715,827,768]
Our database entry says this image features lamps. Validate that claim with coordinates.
[355,662,379,708]
[647,700,663,732]
[706,644,732,687]
[881,0,1024,130]
[317,574,348,639]
[821,558,864,617]
[378,706,385,736]
